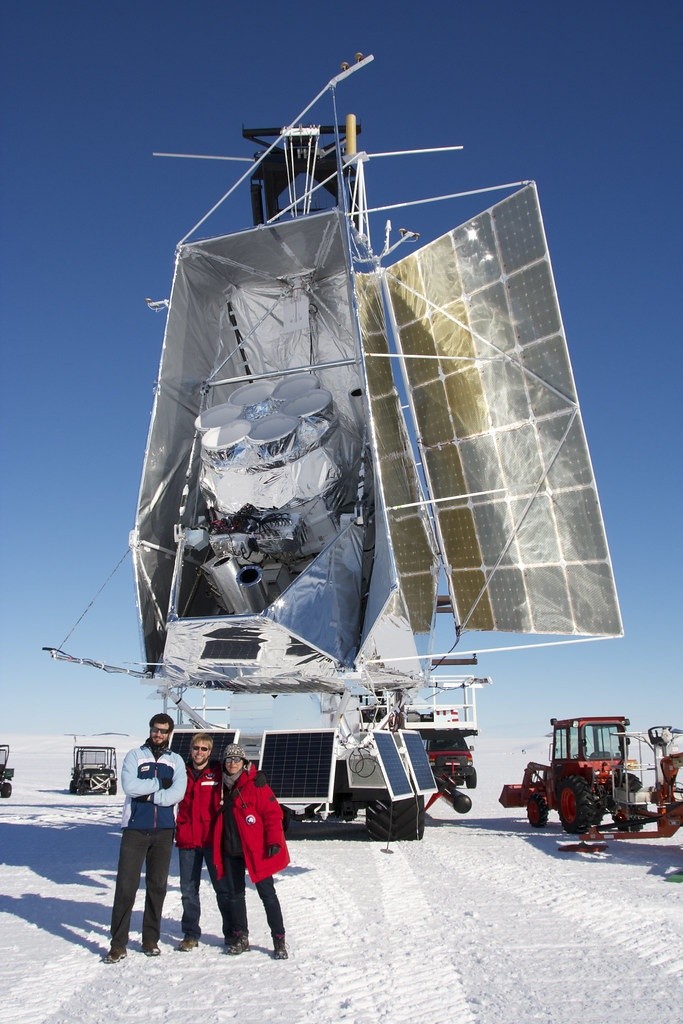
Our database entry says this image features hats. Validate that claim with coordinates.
[220,743,252,805]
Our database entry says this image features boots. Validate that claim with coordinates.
[226,930,250,954]
[273,934,288,960]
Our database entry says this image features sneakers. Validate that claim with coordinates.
[104,946,127,964]
[179,935,198,951]
[225,935,232,945]
[142,942,161,956]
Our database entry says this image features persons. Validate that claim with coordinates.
[175,733,268,952]
[210,743,291,959]
[103,713,188,962]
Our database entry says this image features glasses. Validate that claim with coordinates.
[150,726,170,734]
[193,746,210,751]
[225,757,244,762]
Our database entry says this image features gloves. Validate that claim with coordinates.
[268,844,279,857]
[136,794,150,801]
[161,777,173,790]
[254,772,266,787]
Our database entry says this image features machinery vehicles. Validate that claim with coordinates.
[499,712,650,833]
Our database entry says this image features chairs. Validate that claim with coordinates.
[590,751,614,757]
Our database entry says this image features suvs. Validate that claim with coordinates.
[425,737,478,789]
